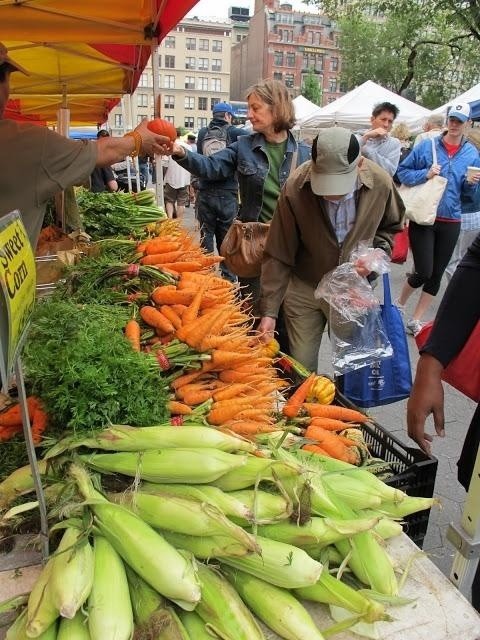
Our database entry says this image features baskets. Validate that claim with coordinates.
[285,373,439,540]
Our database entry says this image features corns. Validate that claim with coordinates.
[0,425,437,640]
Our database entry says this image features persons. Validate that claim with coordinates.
[0,44,172,258]
[89,130,119,193]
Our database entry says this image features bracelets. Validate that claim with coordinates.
[123,130,143,167]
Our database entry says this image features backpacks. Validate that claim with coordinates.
[201,124,233,157]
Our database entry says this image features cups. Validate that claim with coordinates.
[466,166,480,185]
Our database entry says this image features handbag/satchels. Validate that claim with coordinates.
[398,136,448,226]
[333,273,412,409]
[389,218,409,264]
[220,219,270,278]
[415,318,480,405]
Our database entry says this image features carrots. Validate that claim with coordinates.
[125,219,368,465]
[0,394,49,445]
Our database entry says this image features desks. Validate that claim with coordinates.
[252,520,480,639]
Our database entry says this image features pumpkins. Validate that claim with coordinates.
[306,376,335,404]
[147,118,177,145]
[254,338,279,359]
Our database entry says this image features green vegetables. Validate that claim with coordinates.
[78,189,165,238]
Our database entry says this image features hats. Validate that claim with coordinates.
[310,127,361,197]
[213,103,237,119]
[1,42,30,76]
[447,103,472,123]
[97,130,109,137]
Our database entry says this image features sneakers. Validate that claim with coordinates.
[392,297,405,317]
[404,318,426,337]
